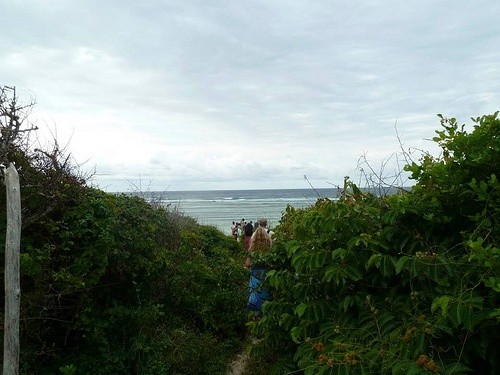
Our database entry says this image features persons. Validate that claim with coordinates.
[245,226,278,320]
[231,216,275,253]
